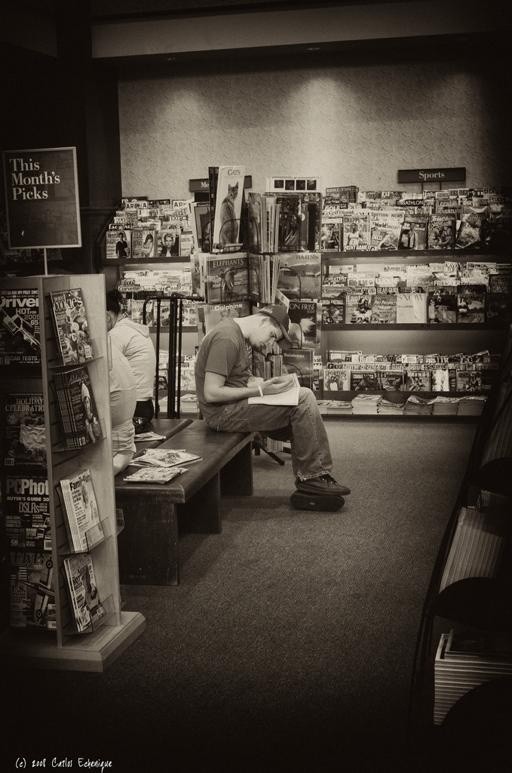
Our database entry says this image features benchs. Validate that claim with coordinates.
[113,418,258,588]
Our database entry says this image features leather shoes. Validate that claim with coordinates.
[290,474,351,511]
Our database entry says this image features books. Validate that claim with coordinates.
[129,429,200,486]
[5,285,112,637]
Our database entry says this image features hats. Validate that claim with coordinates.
[257,305,292,342]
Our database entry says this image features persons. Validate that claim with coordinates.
[190,304,350,512]
[107,342,141,478]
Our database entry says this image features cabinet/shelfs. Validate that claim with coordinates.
[92,197,511,420]
[0,276,130,651]
